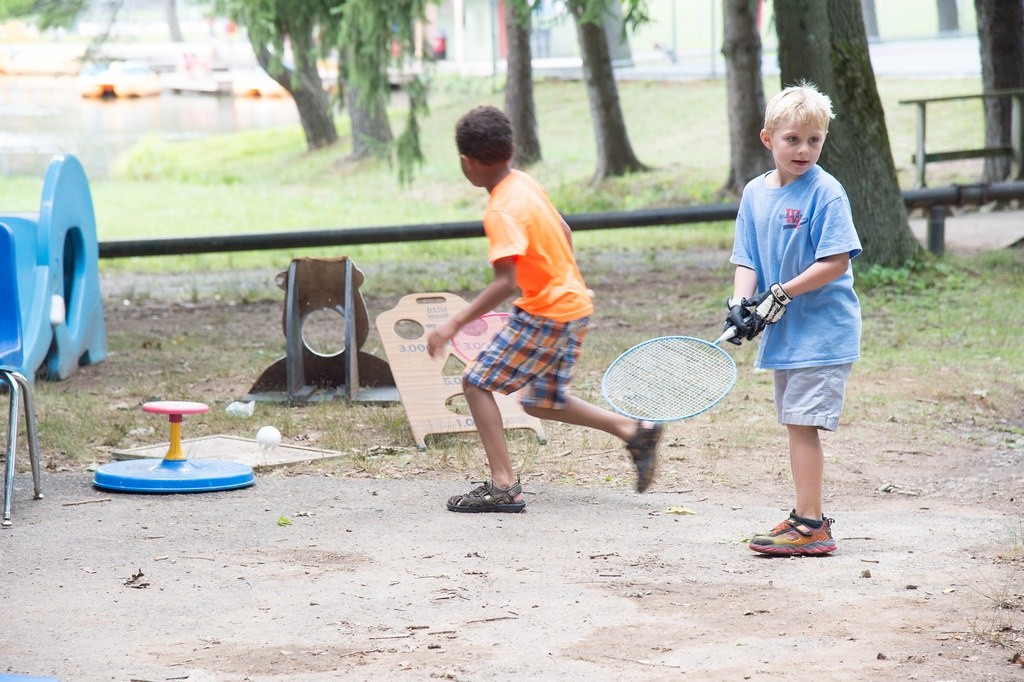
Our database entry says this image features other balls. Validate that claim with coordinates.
[256,426,282,449]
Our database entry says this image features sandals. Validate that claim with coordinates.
[447,480,525,514]
[624,419,662,492]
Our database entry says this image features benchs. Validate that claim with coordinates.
[899,87,1023,212]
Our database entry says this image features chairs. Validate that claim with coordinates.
[0,223,43,527]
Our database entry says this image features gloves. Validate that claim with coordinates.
[741,283,792,341]
[725,297,752,345]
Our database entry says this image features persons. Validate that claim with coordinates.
[427,106,662,514]
[725,79,863,557]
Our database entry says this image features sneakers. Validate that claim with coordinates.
[749,508,836,553]
